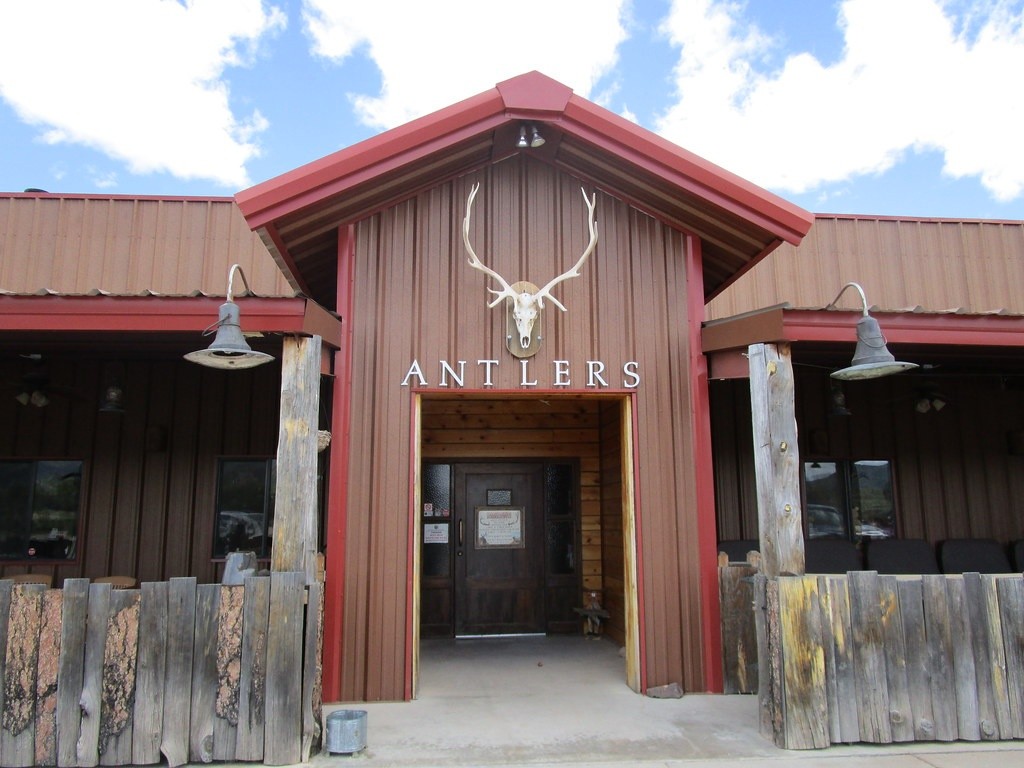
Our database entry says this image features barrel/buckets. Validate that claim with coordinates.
[326,709,367,753]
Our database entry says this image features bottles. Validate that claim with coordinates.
[586,593,600,637]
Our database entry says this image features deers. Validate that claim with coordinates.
[460,182,600,349]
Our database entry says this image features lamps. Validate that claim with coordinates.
[832,390,852,417]
[824,282,920,381]
[99,372,128,414]
[911,372,946,414]
[182,263,275,371]
[514,120,546,148]
[14,370,56,410]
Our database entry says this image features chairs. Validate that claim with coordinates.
[936,539,1013,574]
[2,574,52,589]
[94,576,137,589]
[718,538,760,563]
[859,539,938,574]
[805,534,862,574]
[1008,539,1024,574]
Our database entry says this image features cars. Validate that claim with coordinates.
[802,501,891,543]
